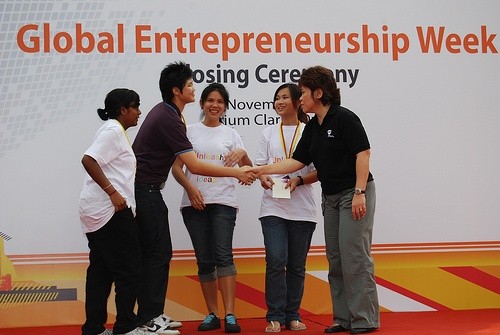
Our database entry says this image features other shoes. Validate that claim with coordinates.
[350,326,378,334]
[324,323,347,332]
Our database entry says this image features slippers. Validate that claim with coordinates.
[286,319,307,329]
[264,320,282,332]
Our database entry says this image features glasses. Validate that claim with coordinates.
[129,104,140,109]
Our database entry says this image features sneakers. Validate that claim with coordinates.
[119,325,160,335]
[197,311,222,330]
[97,328,113,335]
[142,320,181,334]
[225,313,241,332]
[152,314,182,327]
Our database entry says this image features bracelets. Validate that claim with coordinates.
[103,184,112,189]
[108,190,116,196]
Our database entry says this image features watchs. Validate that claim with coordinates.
[297,175,304,186]
[354,188,365,195]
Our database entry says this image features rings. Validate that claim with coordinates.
[360,208,362,211]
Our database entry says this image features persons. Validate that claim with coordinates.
[79,89,160,335]
[238,66,379,334]
[253,83,320,332]
[171,83,253,333]
[126,63,259,335]
